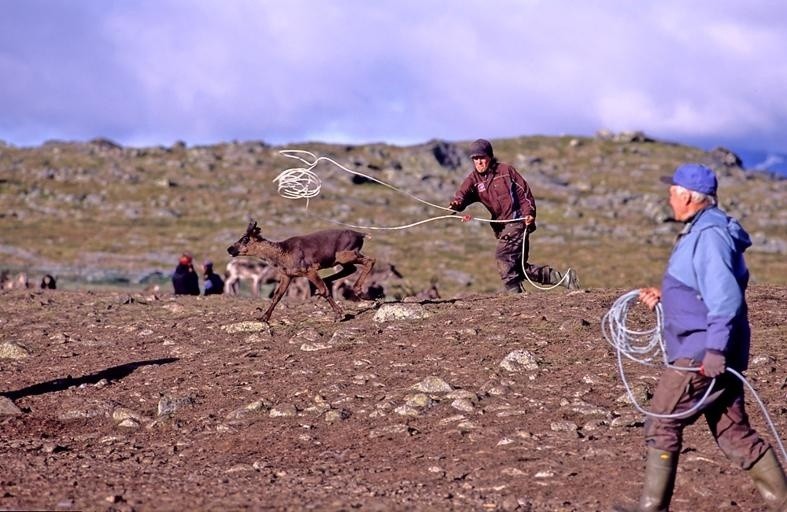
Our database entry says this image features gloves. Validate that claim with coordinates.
[702,351,725,377]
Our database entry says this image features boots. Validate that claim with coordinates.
[747,448,787,512]
[611,447,678,512]
[505,281,521,294]
[549,268,580,292]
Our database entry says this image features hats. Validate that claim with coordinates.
[470,139,493,157]
[180,254,193,265]
[660,163,718,196]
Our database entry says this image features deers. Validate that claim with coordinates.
[223,218,378,322]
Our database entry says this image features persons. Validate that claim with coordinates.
[170,251,199,295]
[40,274,56,290]
[609,162,787,511]
[200,260,225,296]
[446,138,583,294]
